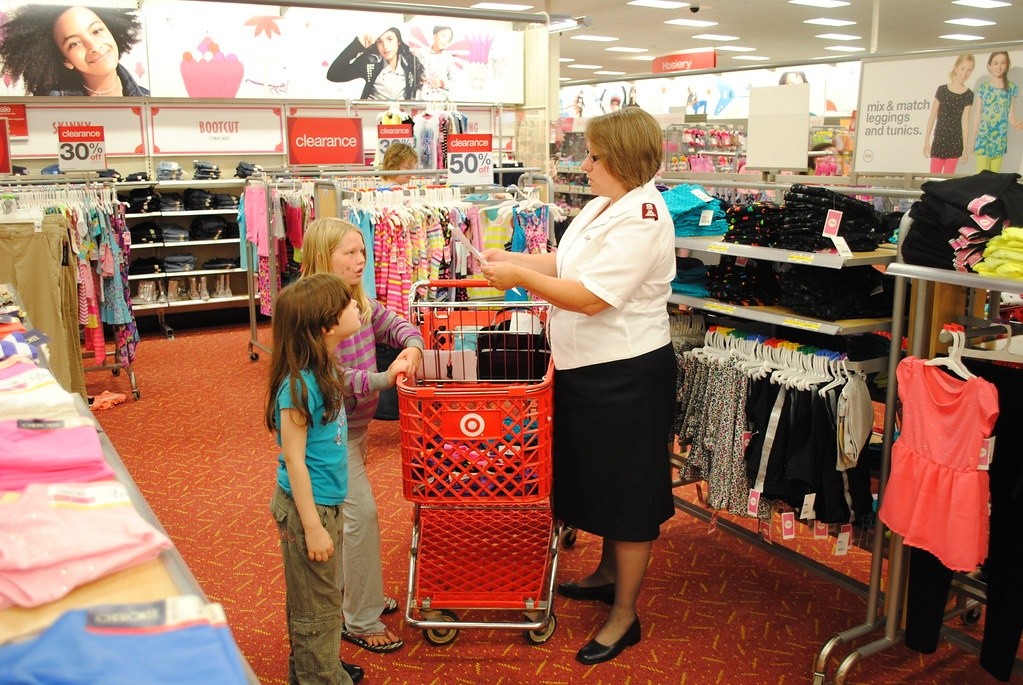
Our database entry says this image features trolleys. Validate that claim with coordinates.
[394,280,578,645]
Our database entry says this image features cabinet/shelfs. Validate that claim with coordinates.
[667,234,913,376]
[553,167,599,216]
[152,172,261,316]
[113,180,169,317]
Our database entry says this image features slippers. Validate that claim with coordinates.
[341,621,403,652]
[379,597,398,614]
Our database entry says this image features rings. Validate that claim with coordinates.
[487,280,492,287]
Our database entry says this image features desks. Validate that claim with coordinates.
[1,283,260,685]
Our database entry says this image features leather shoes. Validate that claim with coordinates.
[576,616,641,664]
[558,582,616,604]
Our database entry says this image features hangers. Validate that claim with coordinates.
[267,178,566,226]
[921,321,1023,381]
[376,99,463,124]
[666,306,867,416]
[0,181,131,232]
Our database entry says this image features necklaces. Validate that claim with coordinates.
[79,76,120,95]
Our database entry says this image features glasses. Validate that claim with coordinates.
[585,149,608,165]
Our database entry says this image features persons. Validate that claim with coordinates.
[377,143,419,187]
[476,105,679,665]
[326,25,466,100]
[969,51,1023,174]
[573,84,640,118]
[923,54,975,174]
[0,5,151,98]
[778,71,817,116]
[799,142,838,176]
[686,87,707,114]
[295,216,425,652]
[261,272,364,685]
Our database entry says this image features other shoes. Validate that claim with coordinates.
[340,660,362,685]
